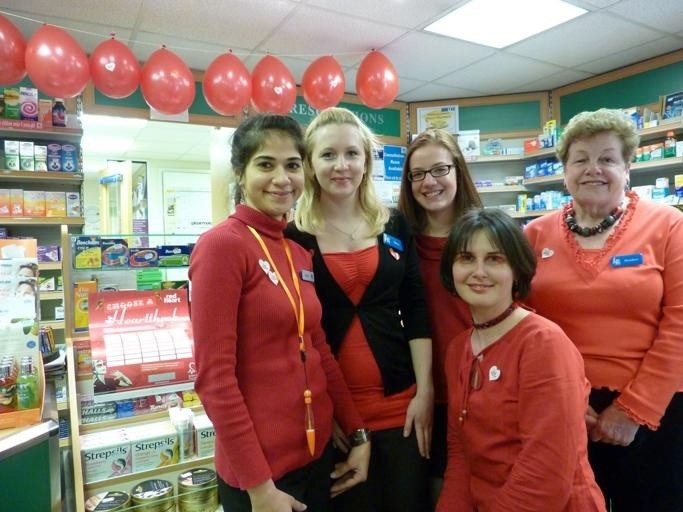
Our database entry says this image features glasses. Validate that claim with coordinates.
[407,164,456,182]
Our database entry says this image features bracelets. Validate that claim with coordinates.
[348,426,371,448]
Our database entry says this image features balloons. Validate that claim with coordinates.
[24,24,90,100]
[140,46,195,115]
[356,49,399,110]
[301,54,346,109]
[250,55,297,113]
[202,51,253,114]
[0,16,29,86]
[88,40,142,100]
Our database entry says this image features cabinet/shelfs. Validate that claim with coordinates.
[520,121,683,217]
[67,339,224,512]
[456,153,522,221]
[0,119,84,331]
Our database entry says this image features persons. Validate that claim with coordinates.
[276,106,438,512]
[433,208,607,512]
[387,130,487,512]
[515,109,682,512]
[187,115,372,512]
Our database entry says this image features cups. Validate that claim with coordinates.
[79,467,220,512]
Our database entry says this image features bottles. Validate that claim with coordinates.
[662,130,676,157]
[0,356,40,412]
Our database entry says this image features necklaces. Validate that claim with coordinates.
[323,213,366,242]
[471,300,522,331]
[565,204,625,236]
